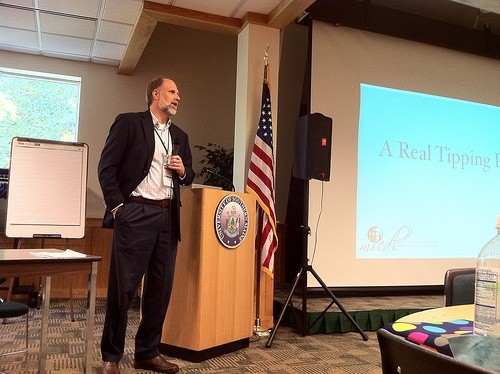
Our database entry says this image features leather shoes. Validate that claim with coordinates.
[134,355,178,373]
[102,361,120,374]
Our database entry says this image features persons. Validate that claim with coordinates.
[96,76,196,374]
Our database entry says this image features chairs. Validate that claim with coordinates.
[377,327,496,374]
[444,267,476,306]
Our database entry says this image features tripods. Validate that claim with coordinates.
[265,180,369,349]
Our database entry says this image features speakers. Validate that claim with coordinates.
[291,113,332,181]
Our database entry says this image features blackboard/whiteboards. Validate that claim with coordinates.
[4,136,91,240]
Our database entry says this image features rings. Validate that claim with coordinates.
[174,162,179,167]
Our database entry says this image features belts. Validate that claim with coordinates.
[127,196,172,208]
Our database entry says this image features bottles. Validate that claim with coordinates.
[472,212,500,338]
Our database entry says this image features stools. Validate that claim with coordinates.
[0,301,29,361]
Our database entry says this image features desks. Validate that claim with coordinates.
[0,248,103,374]
[395,304,474,322]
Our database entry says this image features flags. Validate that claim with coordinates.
[245,81,279,282]
